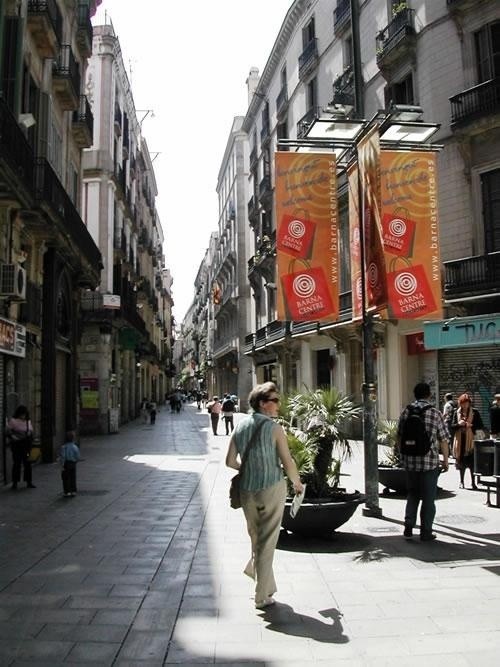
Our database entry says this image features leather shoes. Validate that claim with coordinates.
[458,480,467,489]
[471,482,478,490]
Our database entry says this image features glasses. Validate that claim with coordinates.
[263,396,280,403]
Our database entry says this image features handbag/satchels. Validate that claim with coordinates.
[10,434,33,456]
[63,459,76,470]
[229,474,241,510]
[208,408,212,414]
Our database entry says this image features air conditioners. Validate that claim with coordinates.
[0,262,27,302]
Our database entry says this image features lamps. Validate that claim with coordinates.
[442,316,458,332]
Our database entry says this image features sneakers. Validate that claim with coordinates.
[11,481,18,491]
[27,481,36,489]
[255,592,276,609]
[62,490,79,497]
[403,528,414,540]
[420,533,437,542]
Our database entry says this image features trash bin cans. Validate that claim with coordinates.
[473,438,497,475]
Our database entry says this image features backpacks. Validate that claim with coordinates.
[400,404,435,458]
[448,402,457,421]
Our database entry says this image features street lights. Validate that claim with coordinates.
[277,91,445,518]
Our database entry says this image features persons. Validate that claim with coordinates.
[488,394,500,440]
[225,380,304,609]
[397,382,452,541]
[165,386,237,413]
[450,393,482,491]
[60,431,80,497]
[149,405,156,424]
[221,396,235,435]
[140,398,148,424]
[7,404,37,489]
[208,396,222,435]
[443,392,457,459]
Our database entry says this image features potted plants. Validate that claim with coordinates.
[273,386,367,537]
[377,417,445,497]
